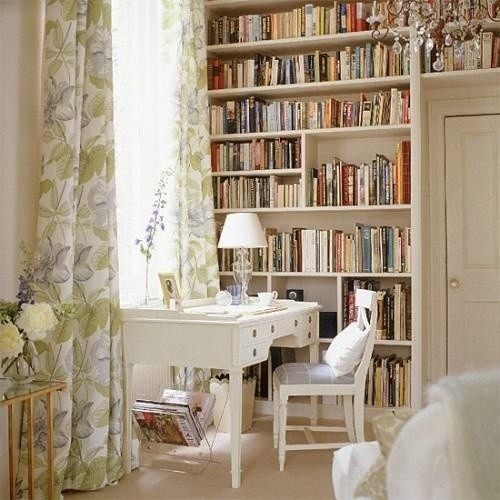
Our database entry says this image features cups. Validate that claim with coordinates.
[169,298,179,310]
[257,291,278,306]
[225,282,242,306]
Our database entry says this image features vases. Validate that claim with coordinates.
[11,353,36,384]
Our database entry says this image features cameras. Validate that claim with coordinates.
[286,289,303,301]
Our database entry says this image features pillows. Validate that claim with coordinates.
[323,320,370,378]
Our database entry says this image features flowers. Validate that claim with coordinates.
[0,274,57,359]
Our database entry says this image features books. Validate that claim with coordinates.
[341,278,411,341]
[213,222,412,274]
[344,351,411,408]
[242,364,269,398]
[209,87,410,136]
[421,0,499,72]
[131,388,215,449]
[213,175,302,208]
[307,141,411,210]
[208,41,409,90]
[208,0,407,45]
[211,136,301,172]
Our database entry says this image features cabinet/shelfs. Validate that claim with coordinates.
[203,2,500,423]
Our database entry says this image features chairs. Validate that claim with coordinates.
[273,288,380,472]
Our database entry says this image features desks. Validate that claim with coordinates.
[1,379,66,500]
[121,297,322,489]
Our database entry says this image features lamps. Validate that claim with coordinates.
[218,213,268,303]
[365,0,490,71]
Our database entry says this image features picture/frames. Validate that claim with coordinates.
[158,273,183,309]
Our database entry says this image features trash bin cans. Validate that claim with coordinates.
[209,372,256,434]
